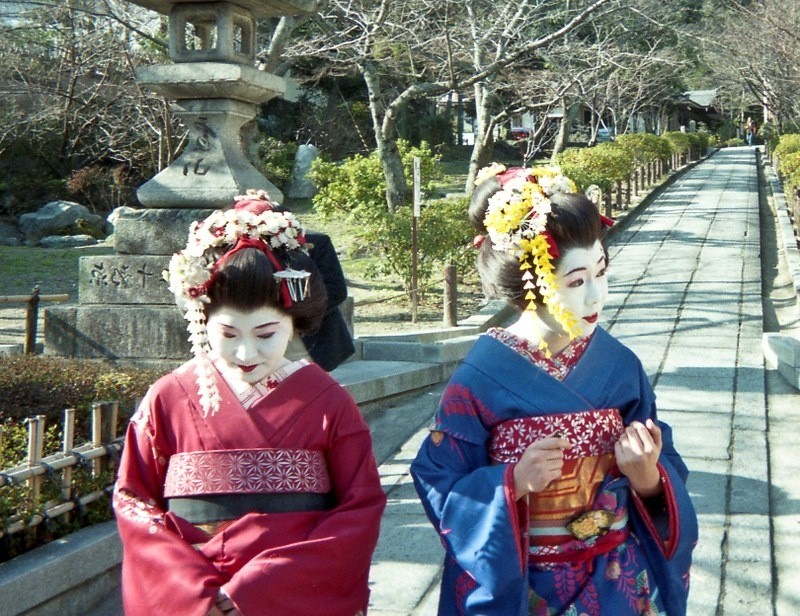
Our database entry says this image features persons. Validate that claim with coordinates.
[410,162,698,616]
[113,188,387,616]
[744,117,756,147]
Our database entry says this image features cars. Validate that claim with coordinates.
[511,127,533,140]
